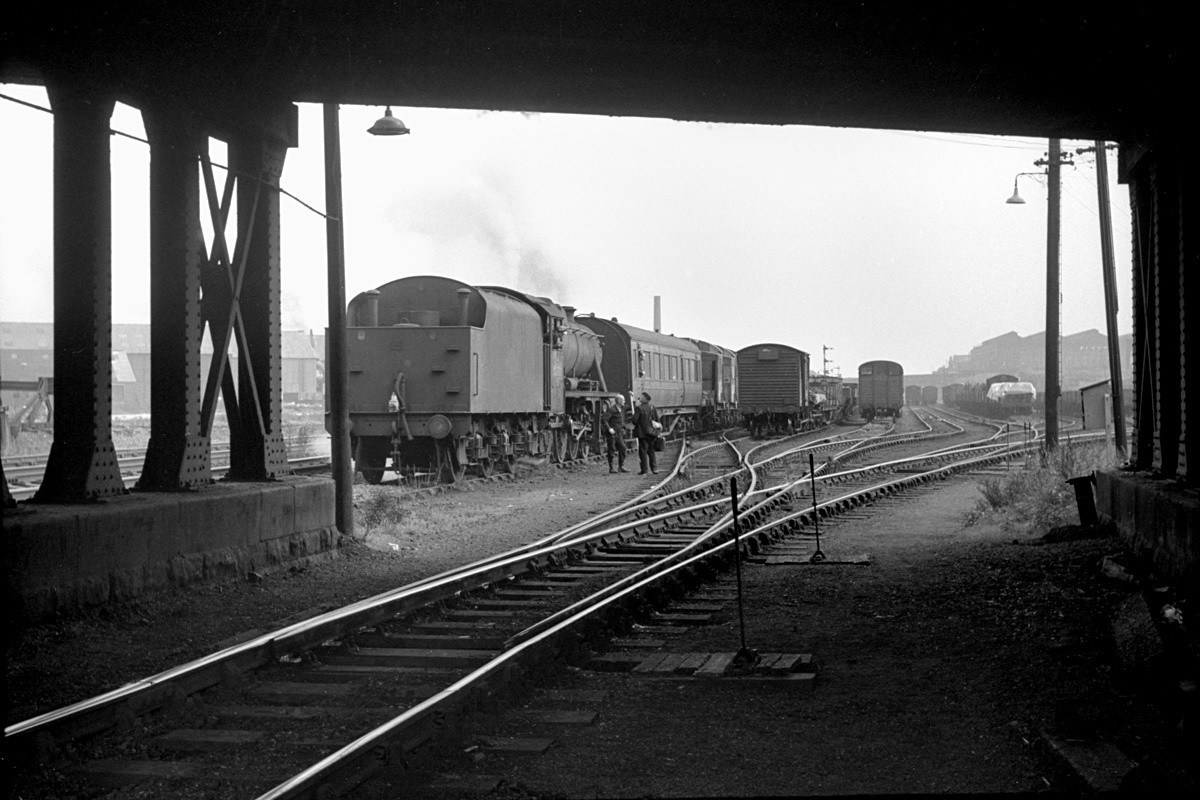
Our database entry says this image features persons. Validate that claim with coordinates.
[601,394,629,473]
[633,392,662,475]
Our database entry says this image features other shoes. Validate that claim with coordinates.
[609,466,618,473]
[653,470,660,474]
[618,466,632,472]
[637,471,649,476]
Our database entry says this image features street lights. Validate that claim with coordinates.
[1006,170,1061,454]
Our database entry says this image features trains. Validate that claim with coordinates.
[942,374,1036,422]
[325,276,738,489]
[858,360,903,423]
[904,385,938,407]
[735,343,852,442]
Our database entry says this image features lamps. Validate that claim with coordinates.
[366,106,410,136]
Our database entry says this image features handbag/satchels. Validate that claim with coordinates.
[647,419,664,437]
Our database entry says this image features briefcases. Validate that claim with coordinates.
[655,435,667,453]
